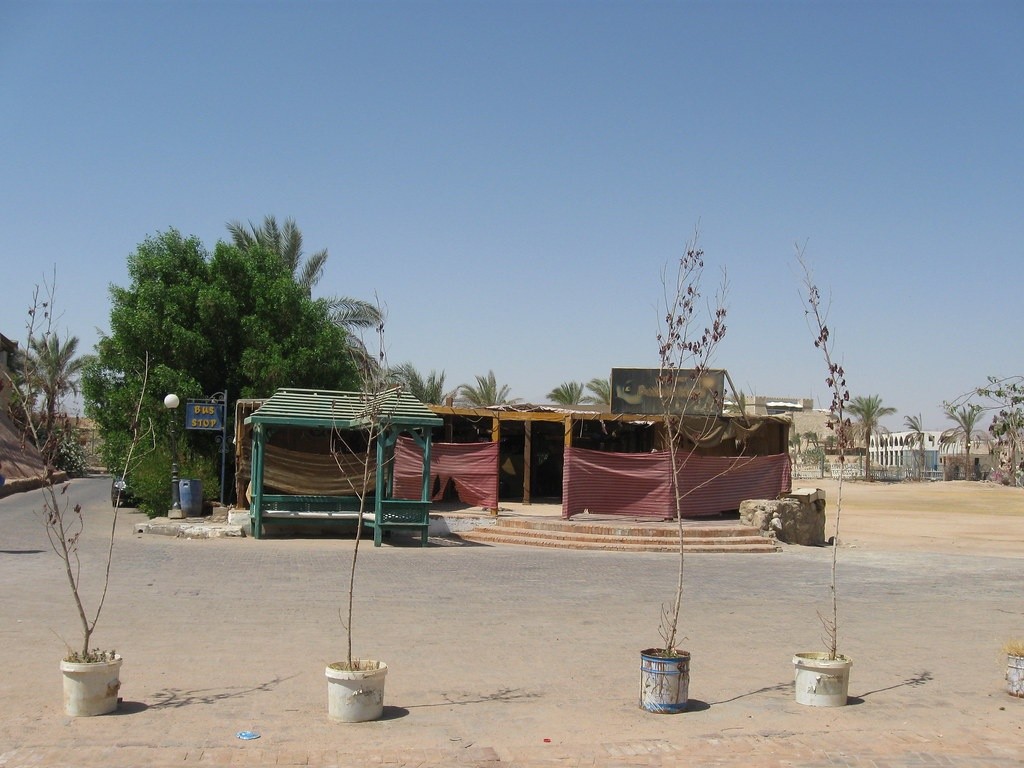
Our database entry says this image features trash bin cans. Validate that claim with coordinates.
[179,478,203,516]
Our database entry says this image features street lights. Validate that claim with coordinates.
[164,394,185,518]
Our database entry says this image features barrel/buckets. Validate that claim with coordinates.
[179,479,203,517]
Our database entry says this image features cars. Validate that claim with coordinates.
[112,473,141,508]
